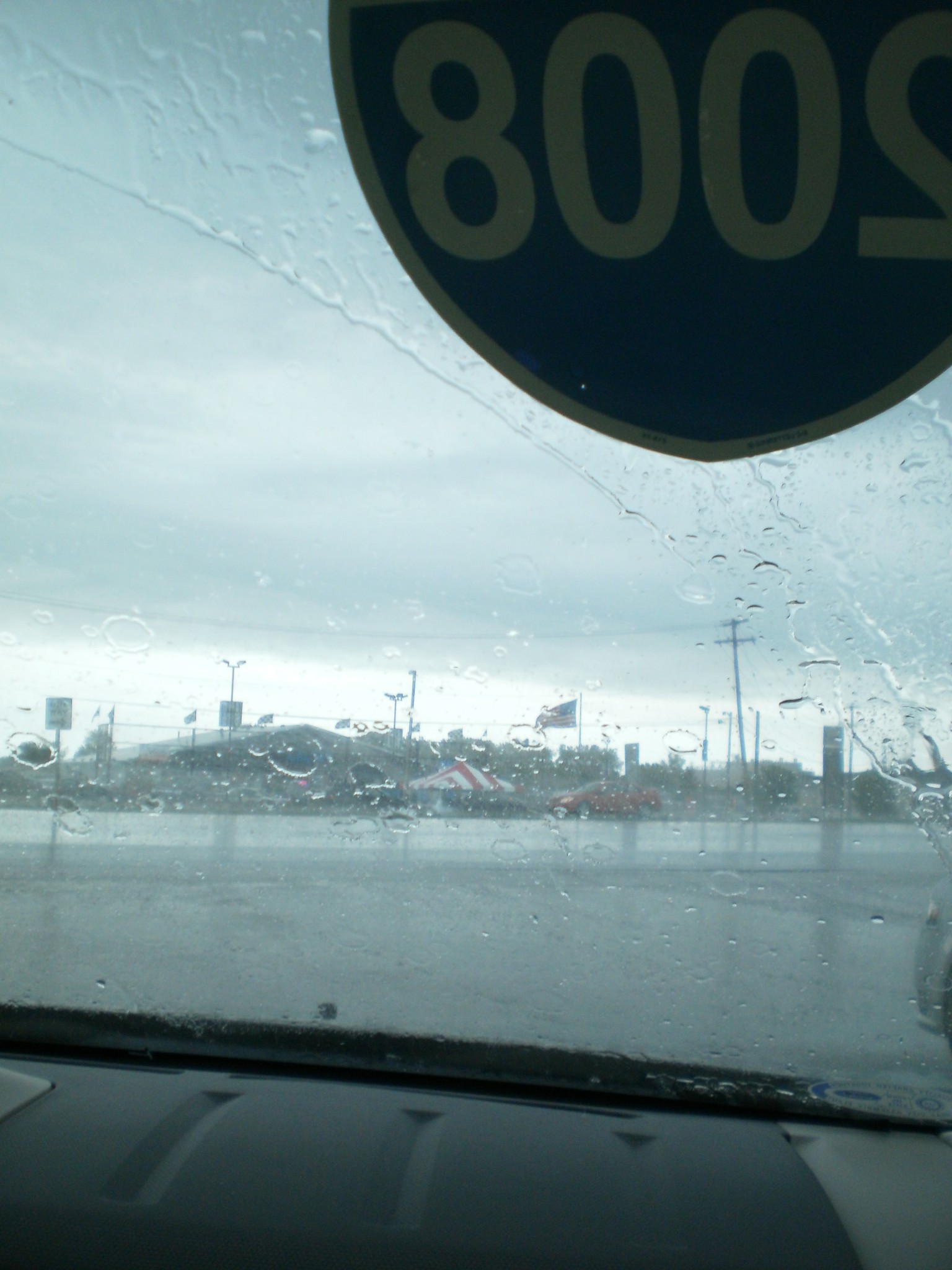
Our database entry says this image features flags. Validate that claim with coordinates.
[536,699,577,728]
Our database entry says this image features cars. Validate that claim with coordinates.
[547,780,663,818]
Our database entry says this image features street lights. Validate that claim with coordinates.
[698,705,710,793]
[722,711,732,788]
[408,670,417,742]
[222,659,246,782]
[385,692,408,759]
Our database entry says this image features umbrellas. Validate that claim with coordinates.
[410,759,523,792]
